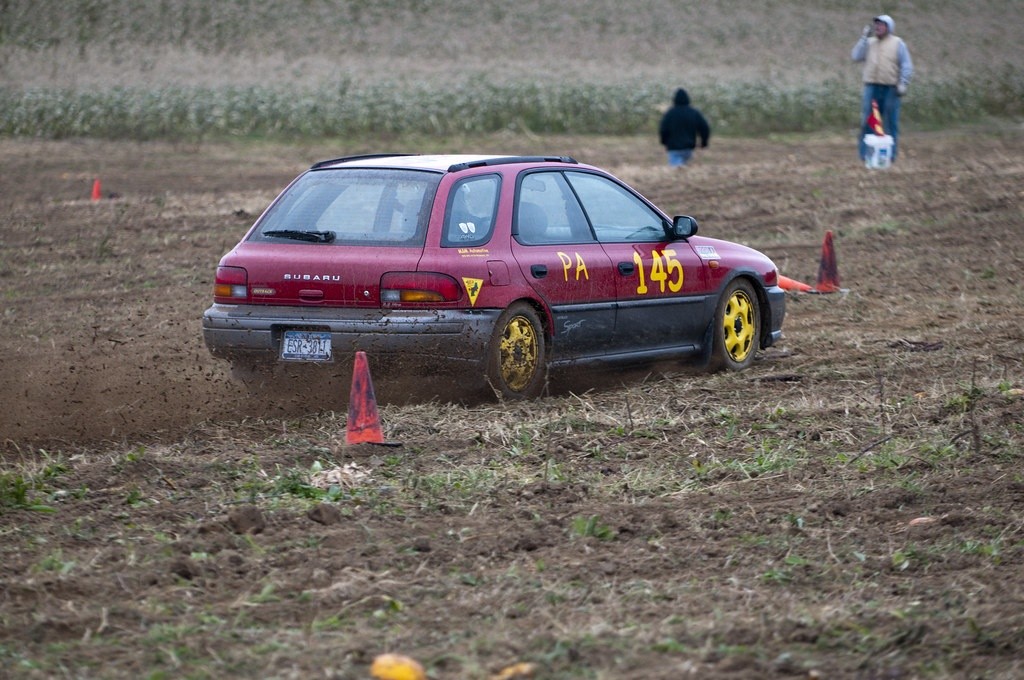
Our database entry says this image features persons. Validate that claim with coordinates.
[851,15,914,162]
[658,87,711,167]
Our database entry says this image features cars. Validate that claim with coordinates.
[201,151,790,408]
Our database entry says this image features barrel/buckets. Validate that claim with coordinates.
[864,133,893,169]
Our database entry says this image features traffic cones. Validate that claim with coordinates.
[811,231,842,294]
[341,349,392,443]
[778,273,814,294]
[91,180,102,201]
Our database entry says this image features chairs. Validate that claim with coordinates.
[400,199,466,234]
[519,202,549,242]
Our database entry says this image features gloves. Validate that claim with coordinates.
[861,25,871,40]
[896,84,907,95]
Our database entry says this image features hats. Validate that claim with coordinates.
[873,15,894,32]
